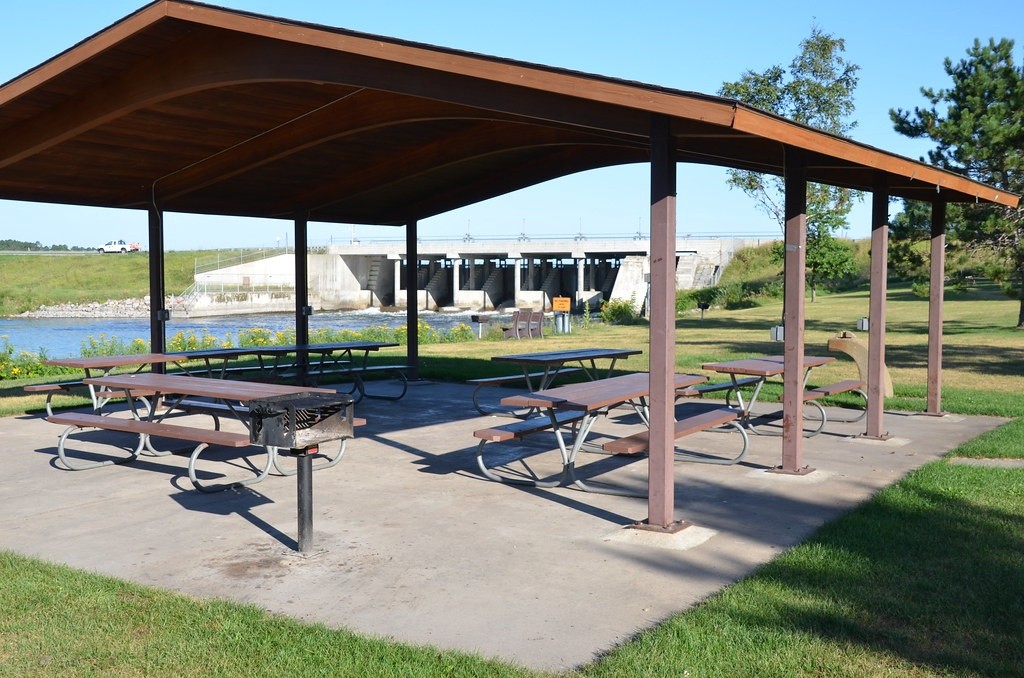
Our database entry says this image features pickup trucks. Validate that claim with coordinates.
[95,239,138,254]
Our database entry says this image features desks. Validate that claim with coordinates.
[43,341,408,492]
[472,348,837,501]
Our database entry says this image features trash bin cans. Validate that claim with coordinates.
[555,312,571,335]
[856,316,869,332]
[770,325,784,342]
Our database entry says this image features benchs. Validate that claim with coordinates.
[46,412,251,447]
[777,380,866,401]
[676,377,767,396]
[275,366,414,379]
[467,368,582,383]
[473,410,597,441]
[24,381,87,391]
[240,361,351,373]
[161,398,367,427]
[601,407,750,454]
[166,368,240,375]
[96,376,269,397]
